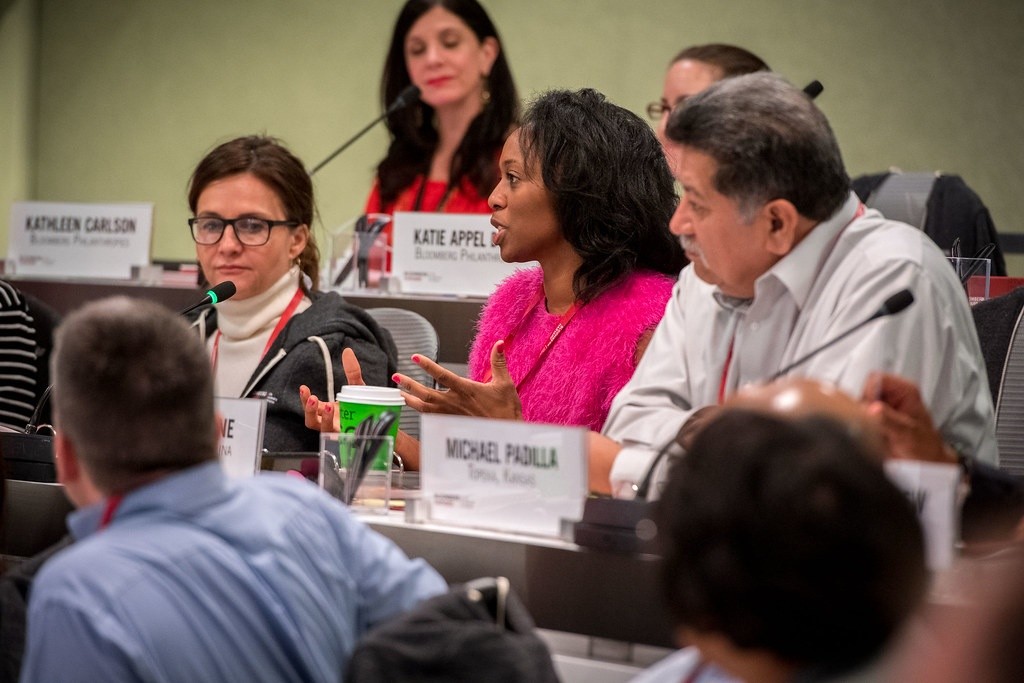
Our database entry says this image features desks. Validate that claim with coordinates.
[0,276,1024,403]
[0,468,678,651]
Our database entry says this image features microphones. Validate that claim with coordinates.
[309,85,423,176]
[0,281,236,484]
[803,80,824,99]
[575,287,915,552]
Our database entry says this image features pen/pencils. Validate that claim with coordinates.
[342,412,396,502]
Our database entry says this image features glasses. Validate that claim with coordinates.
[186,214,302,248]
[644,101,674,122]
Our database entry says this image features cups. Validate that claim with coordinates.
[336,385,406,473]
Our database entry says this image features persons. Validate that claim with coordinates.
[0,278,36,429]
[657,408,1024,683]
[364,0,522,285]
[300,87,691,495]
[179,137,399,470]
[21,295,449,683]
[662,44,772,182]
[587,73,1000,502]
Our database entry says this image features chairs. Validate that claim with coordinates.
[995,307,1024,476]
[25,292,62,436]
[366,307,443,442]
